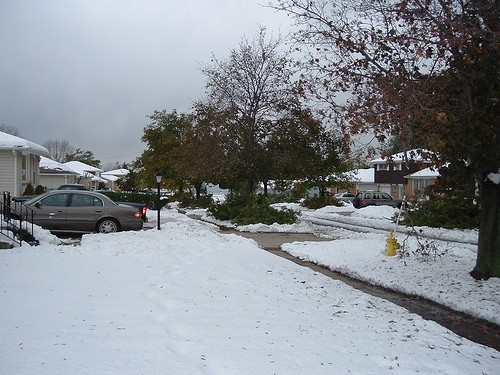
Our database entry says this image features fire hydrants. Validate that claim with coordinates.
[384,232,401,256]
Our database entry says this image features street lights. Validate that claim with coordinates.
[154,169,164,230]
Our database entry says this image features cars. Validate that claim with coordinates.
[334,192,356,203]
[9,183,147,237]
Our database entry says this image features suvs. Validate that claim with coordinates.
[353,190,407,209]
